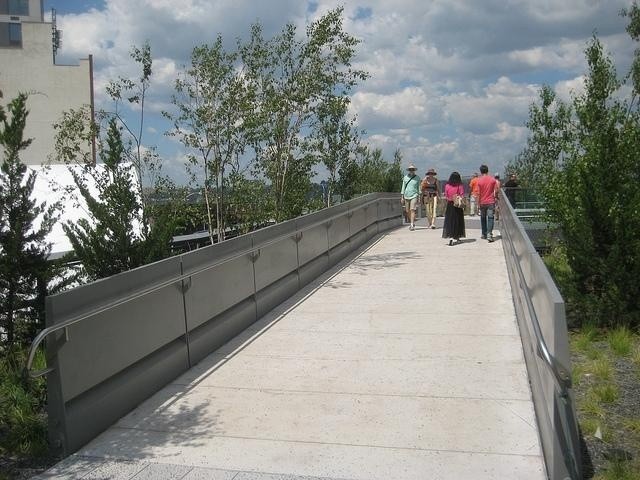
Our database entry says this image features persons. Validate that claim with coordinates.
[441,172,466,246]
[420,169,441,228]
[469,173,481,216]
[400,165,422,231]
[477,164,498,241]
[495,173,501,220]
[504,174,522,209]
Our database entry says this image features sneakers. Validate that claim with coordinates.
[428,224,436,228]
[481,235,494,242]
[449,239,461,245]
[409,224,415,231]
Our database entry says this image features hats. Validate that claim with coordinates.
[406,164,417,171]
[426,169,436,175]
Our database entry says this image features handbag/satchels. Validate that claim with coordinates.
[454,194,466,208]
